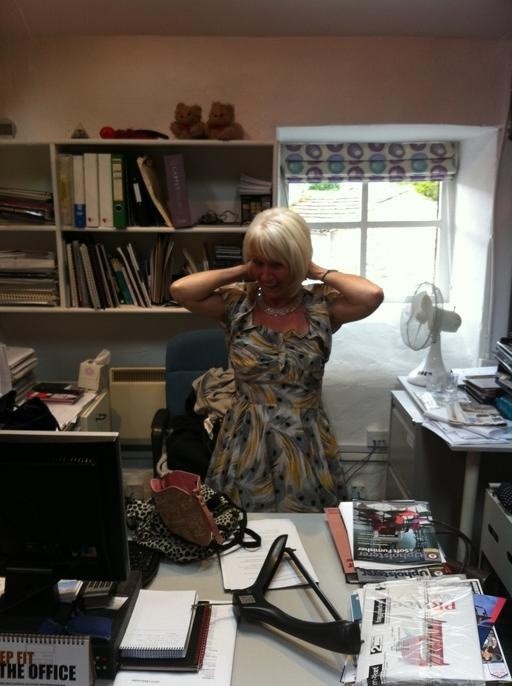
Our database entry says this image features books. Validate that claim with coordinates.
[23,382,85,404]
[464,337,512,421]
[0,345,37,402]
[238,173,273,196]
[0,187,55,225]
[341,574,512,686]
[0,248,60,307]
[324,500,451,583]
[57,152,195,230]
[118,590,211,673]
[63,232,246,310]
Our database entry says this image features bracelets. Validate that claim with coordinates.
[321,270,337,281]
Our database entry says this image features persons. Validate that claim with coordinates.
[172,207,384,514]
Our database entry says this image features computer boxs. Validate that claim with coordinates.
[0,571,142,679]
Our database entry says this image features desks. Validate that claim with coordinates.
[392,383,511,564]
[116,509,452,686]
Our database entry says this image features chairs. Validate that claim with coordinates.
[152,328,249,476]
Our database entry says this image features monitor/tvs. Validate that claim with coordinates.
[1,429,130,617]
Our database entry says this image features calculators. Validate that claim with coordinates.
[80,579,116,608]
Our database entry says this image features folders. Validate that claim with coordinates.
[56,153,128,227]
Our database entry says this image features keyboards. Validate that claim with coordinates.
[128,543,160,587]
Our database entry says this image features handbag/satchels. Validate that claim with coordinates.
[126,470,261,563]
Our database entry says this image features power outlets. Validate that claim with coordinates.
[367,428,390,450]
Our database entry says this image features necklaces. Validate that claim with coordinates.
[257,288,304,317]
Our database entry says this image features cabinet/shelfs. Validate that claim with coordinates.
[480,487,511,599]
[1,137,276,314]
[79,393,114,429]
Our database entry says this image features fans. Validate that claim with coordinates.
[401,279,462,392]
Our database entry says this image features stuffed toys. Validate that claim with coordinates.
[169,101,204,140]
[204,100,243,141]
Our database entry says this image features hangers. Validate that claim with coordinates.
[186,532,365,660]
